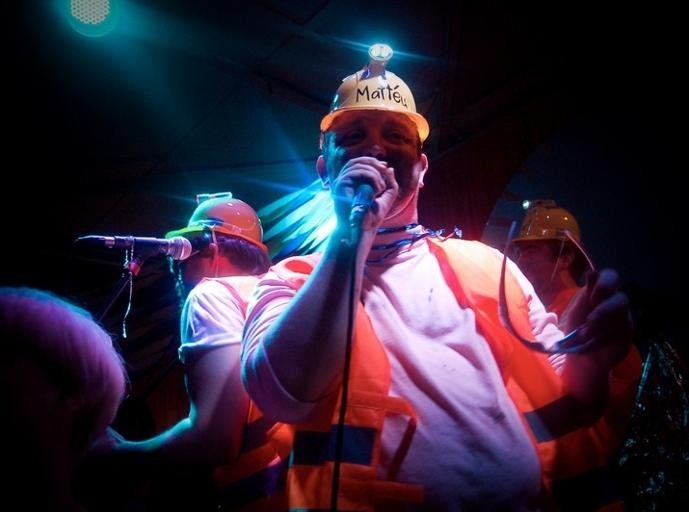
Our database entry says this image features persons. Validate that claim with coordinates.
[240,43,635,512]
[513,199,642,512]
[0,285,125,512]
[89,192,283,512]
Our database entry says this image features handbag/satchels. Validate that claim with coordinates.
[617,337,688,510]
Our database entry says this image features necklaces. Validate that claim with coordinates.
[367,246,401,263]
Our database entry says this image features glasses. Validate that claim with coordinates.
[500,222,601,355]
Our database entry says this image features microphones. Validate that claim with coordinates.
[348,182,375,247]
[78,236,193,261]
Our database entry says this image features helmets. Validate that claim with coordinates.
[165,196,269,255]
[320,44,431,141]
[512,198,581,246]
